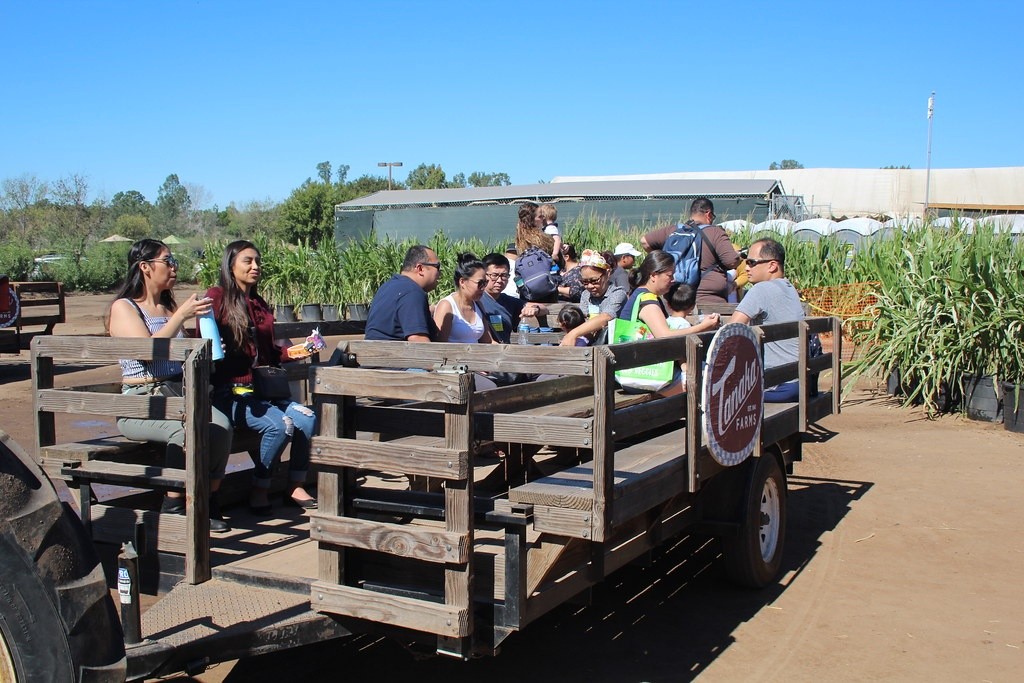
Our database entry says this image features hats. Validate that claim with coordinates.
[506,242,516,251]
[613,242,642,257]
[579,249,611,271]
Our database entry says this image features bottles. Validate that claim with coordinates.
[518,317,530,344]
[198,296,224,361]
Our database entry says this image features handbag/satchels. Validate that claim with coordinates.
[607,292,674,392]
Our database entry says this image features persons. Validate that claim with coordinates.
[618,250,720,398]
[432,251,511,388]
[359,246,496,478]
[665,284,715,394]
[609,243,643,300]
[195,240,323,515]
[640,198,741,315]
[557,242,585,303]
[506,243,516,254]
[536,249,627,382]
[539,204,565,285]
[726,237,812,403]
[514,202,566,303]
[478,252,549,384]
[104,240,233,532]
[557,305,593,346]
[728,250,752,303]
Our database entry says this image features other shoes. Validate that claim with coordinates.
[209,516,231,532]
[161,496,187,515]
[284,494,319,509]
[254,499,273,515]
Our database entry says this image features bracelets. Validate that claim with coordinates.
[531,305,540,315]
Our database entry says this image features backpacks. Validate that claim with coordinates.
[515,243,560,302]
[664,222,722,291]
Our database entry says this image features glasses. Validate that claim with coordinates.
[706,211,717,220]
[415,262,440,270]
[144,256,180,271]
[469,278,489,289]
[744,258,780,268]
[486,273,510,281]
[581,271,605,286]
